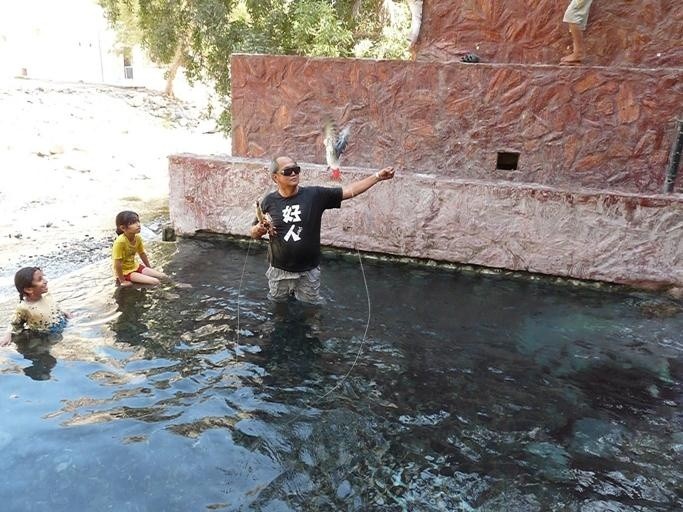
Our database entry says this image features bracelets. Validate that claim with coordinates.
[375,172,382,181]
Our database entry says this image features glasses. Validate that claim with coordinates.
[275,166,299,175]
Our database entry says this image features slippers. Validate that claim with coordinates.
[462,54,478,62]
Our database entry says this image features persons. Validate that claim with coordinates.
[251,156,396,303]
[112,211,169,287]
[559,0,592,66]
[0,267,73,347]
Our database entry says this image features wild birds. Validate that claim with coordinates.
[323,113,352,183]
